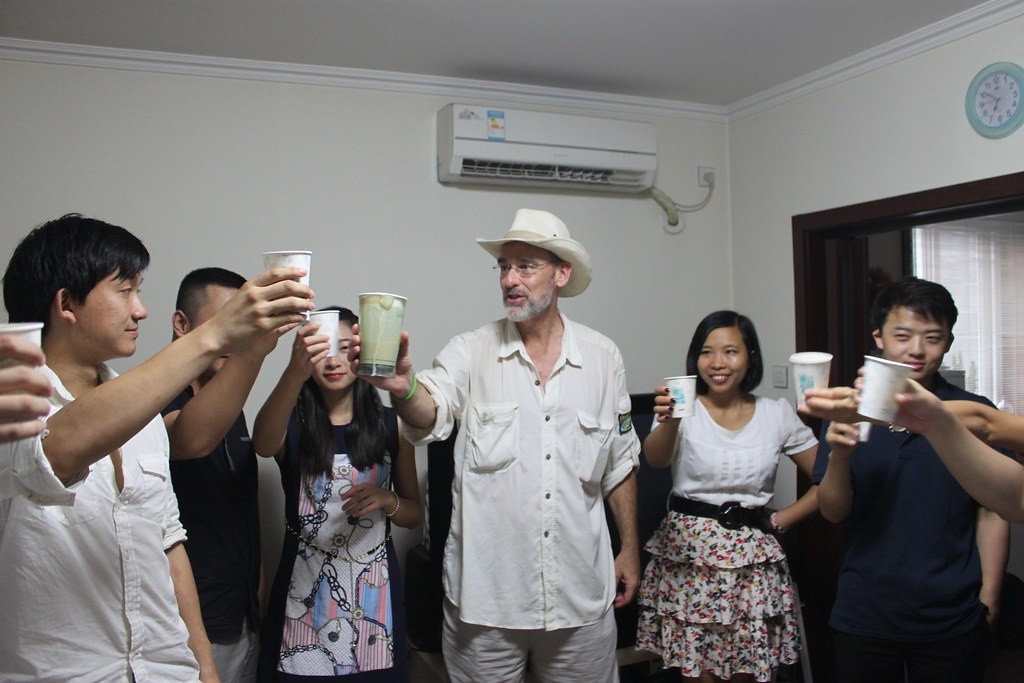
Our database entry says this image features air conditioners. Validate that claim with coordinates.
[437,103,659,193]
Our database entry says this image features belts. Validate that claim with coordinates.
[670,494,779,534]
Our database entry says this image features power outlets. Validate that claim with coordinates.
[698,167,715,187]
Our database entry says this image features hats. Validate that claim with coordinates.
[476,209,592,298]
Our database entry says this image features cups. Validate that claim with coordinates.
[790,352,833,410]
[846,422,872,443]
[1,321,45,394]
[857,355,915,424]
[357,292,409,379]
[310,310,341,358]
[262,251,312,322]
[664,375,698,417]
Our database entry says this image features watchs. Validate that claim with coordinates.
[888,422,909,435]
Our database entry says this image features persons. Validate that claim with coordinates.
[797,385,1024,452]
[812,275,1010,683]
[644,310,819,683]
[253,307,422,683]
[852,366,1024,524]
[0,334,54,443]
[0,213,315,683]
[160,266,303,683]
[348,208,642,683]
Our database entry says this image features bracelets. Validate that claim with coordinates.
[390,373,417,404]
[382,491,400,517]
[770,511,788,536]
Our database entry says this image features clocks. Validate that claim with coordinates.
[965,62,1023,139]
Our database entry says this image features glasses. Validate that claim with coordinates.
[492,258,561,278]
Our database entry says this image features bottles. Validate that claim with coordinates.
[996,395,1014,413]
